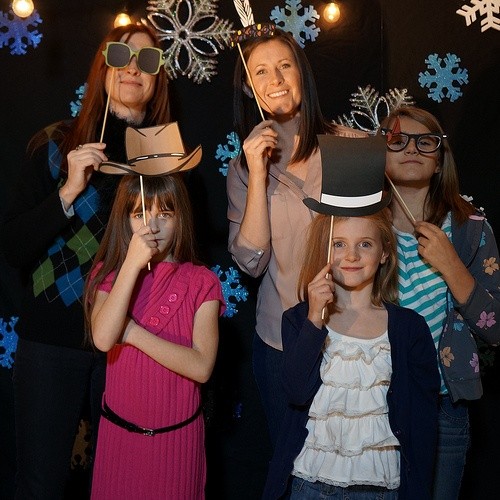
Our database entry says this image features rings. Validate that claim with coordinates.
[76,144,82,151]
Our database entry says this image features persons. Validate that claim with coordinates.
[225,26,370,500]
[83,169,224,500]
[376,106,500,499]
[280,200,443,499]
[11,22,190,500]
